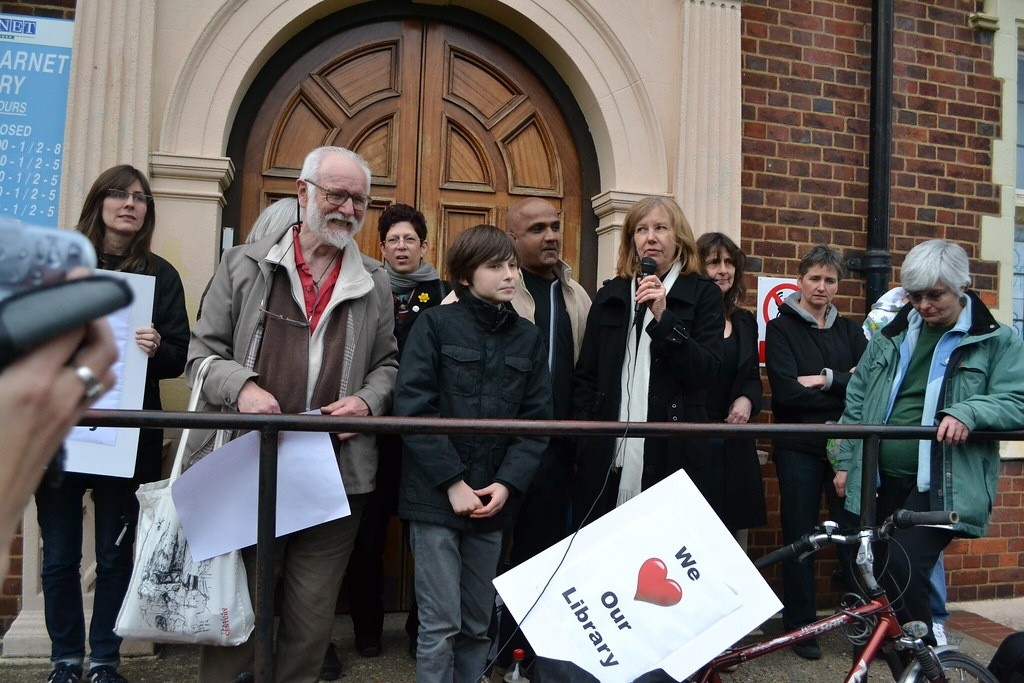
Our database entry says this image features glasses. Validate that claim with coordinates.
[383,236,420,246]
[259,269,319,328]
[107,189,153,205]
[908,287,948,303]
[304,179,372,211]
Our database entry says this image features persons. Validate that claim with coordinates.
[182,145,400,683]
[833,236,1024,669]
[394,229,553,683]
[573,197,727,530]
[764,243,869,658]
[694,232,769,671]
[350,203,451,659]
[34,163,191,683]
[0,260,119,599]
[439,196,593,676]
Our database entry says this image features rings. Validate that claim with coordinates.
[67,357,98,388]
[151,343,157,351]
[85,380,104,400]
[653,280,661,288]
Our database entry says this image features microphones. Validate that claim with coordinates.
[635,256,657,312]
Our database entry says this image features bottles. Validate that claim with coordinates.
[502,649,533,683]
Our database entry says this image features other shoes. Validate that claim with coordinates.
[792,639,822,659]
[716,647,738,672]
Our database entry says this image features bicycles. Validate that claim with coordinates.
[513,508,1001,683]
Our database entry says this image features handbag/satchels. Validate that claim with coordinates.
[113,356,256,646]
[825,413,845,473]
[514,655,690,683]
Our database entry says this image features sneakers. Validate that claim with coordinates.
[356,634,380,656]
[47,661,83,683]
[320,642,341,679]
[86,664,127,683]
[410,640,417,658]
[931,622,948,646]
